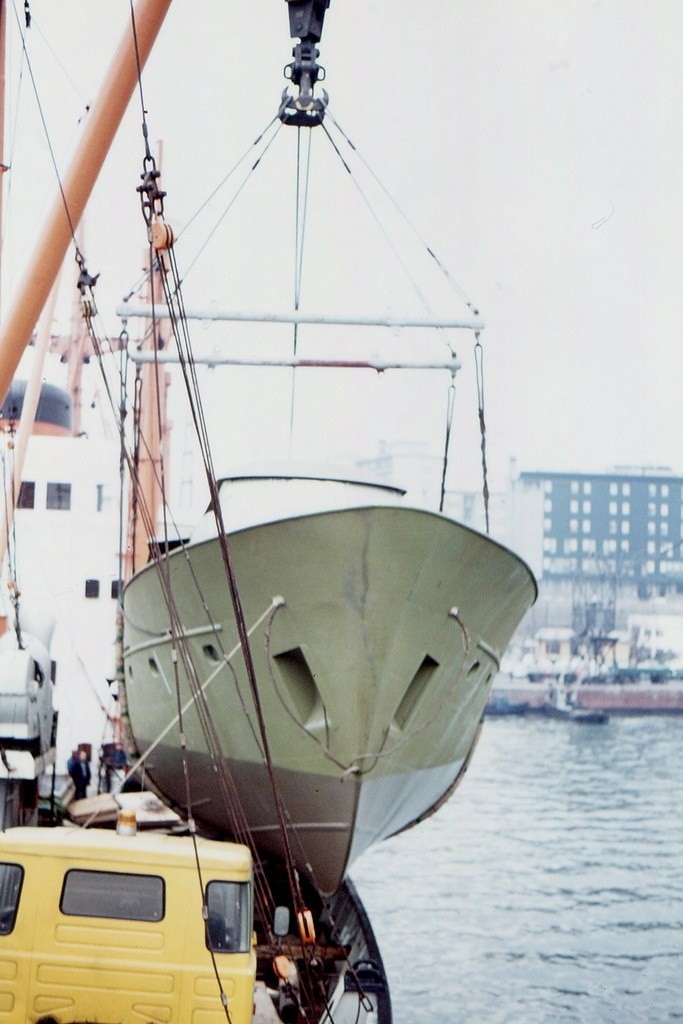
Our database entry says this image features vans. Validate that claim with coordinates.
[0,812,257,1024]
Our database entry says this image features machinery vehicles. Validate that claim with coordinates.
[0,0,328,781]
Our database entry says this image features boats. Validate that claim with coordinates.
[112,468,536,900]
[478,613,683,719]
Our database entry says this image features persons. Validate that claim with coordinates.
[67,743,128,800]
